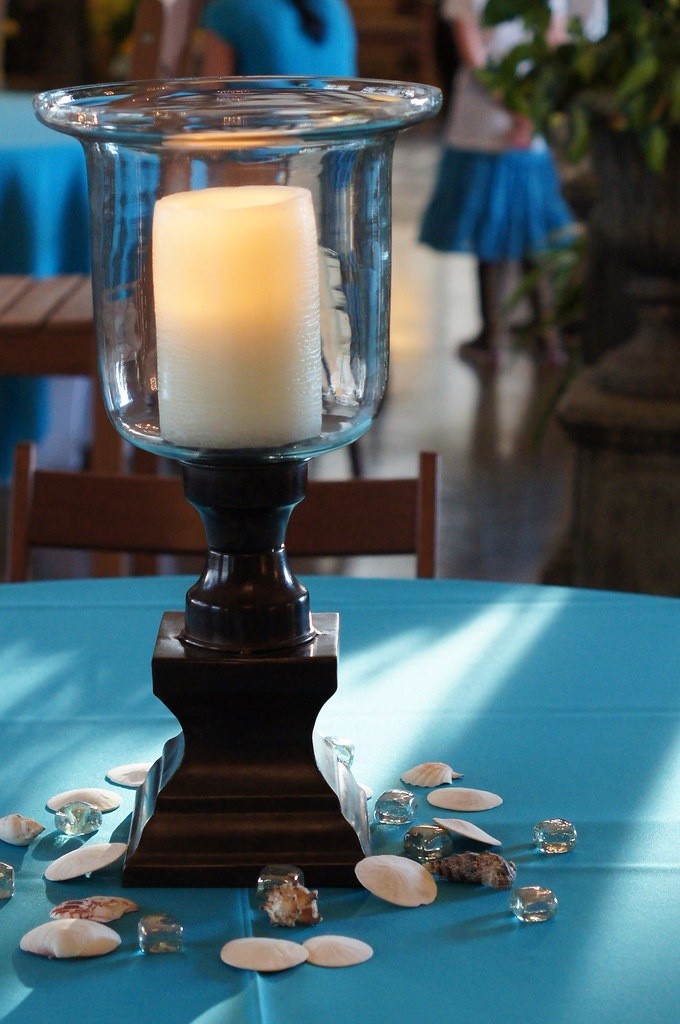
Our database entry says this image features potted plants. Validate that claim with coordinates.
[470,0,680,397]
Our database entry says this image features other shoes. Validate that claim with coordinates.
[462,333,509,368]
[532,337,567,369]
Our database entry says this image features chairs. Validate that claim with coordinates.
[0,0,234,579]
[5,441,439,581]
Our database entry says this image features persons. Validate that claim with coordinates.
[179,0,361,91]
[412,0,613,360]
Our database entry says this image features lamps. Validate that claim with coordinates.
[35,75,443,886]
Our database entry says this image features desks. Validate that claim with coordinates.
[0,577,679,1023]
